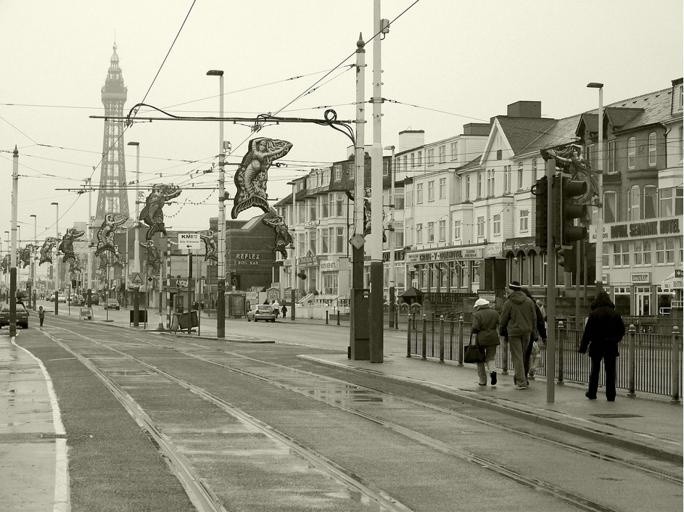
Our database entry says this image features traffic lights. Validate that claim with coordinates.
[552,172,589,252]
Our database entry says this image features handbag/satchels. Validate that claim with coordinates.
[464,332,485,364]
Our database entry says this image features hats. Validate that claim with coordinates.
[508,281,522,291]
[474,299,489,307]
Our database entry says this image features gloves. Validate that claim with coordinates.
[499,327,505,336]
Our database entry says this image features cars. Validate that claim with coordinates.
[103,297,119,310]
[0,303,28,330]
[43,290,100,307]
[245,304,275,322]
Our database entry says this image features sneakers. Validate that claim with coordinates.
[585,391,596,399]
[491,371,497,385]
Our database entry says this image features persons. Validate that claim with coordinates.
[202,231,217,258]
[15,298,24,306]
[500,281,538,389]
[536,299,546,320]
[143,184,181,240]
[1,228,84,273]
[38,306,44,327]
[17,289,21,298]
[350,189,387,241]
[95,215,128,256]
[264,217,289,257]
[521,288,546,377]
[233,138,290,219]
[265,298,287,318]
[470,298,500,386]
[142,243,160,267]
[577,294,625,401]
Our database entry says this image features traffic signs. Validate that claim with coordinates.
[177,233,201,251]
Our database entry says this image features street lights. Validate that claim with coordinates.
[49,202,59,316]
[29,214,37,312]
[15,226,21,289]
[285,182,296,322]
[205,69,228,341]
[584,81,606,300]
[81,177,94,319]
[3,229,9,304]
[383,144,397,329]
[126,140,142,328]
